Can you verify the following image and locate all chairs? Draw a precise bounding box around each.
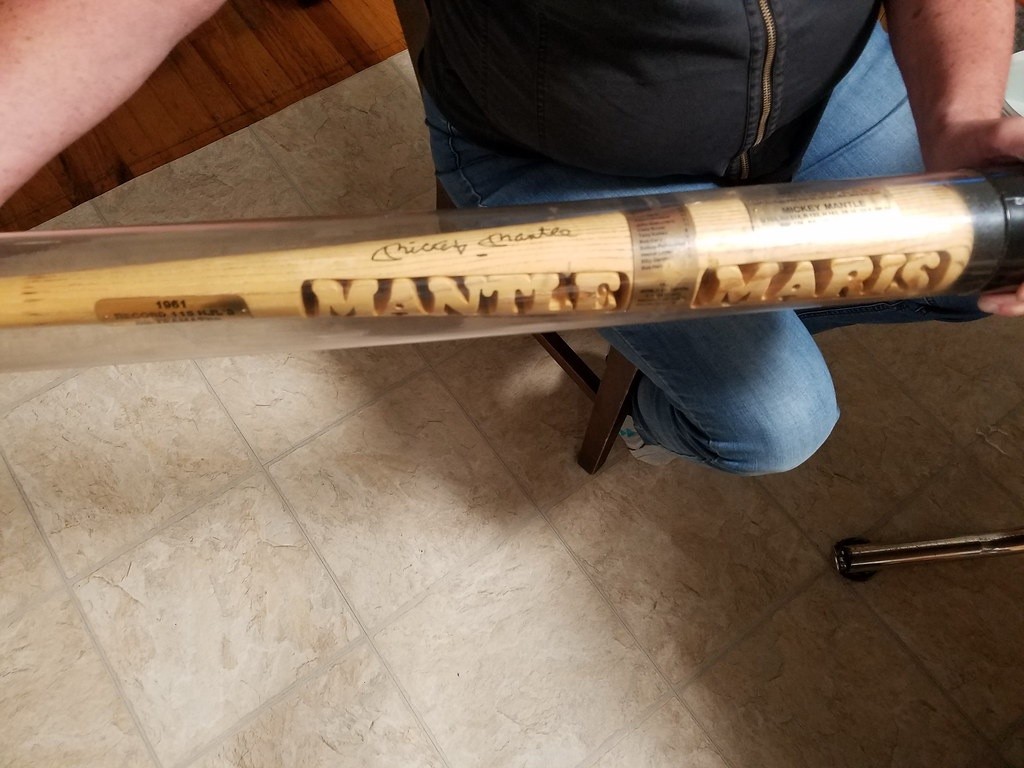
[393,0,646,475]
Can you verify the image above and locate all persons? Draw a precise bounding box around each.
[0,0,1024,476]
